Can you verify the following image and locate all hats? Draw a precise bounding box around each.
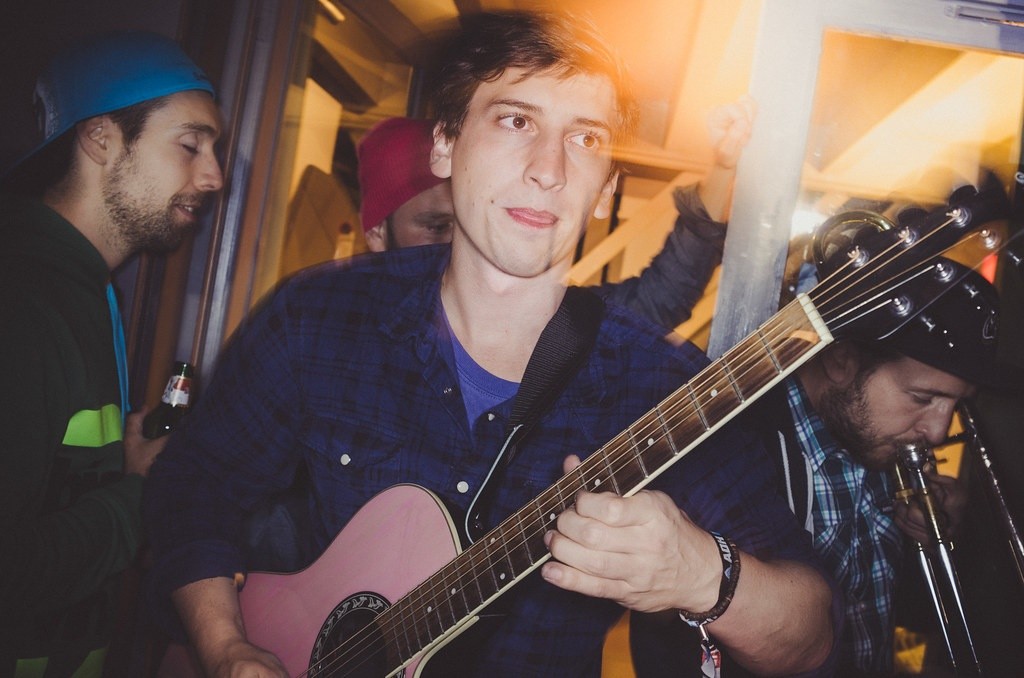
[2,28,218,178]
[850,254,1017,397]
[358,115,452,234]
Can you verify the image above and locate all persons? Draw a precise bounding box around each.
[356,100,754,333]
[761,248,1000,677]
[0,32,225,678]
[140,8,861,678]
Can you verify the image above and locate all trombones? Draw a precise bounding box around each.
[812,210,1023,678]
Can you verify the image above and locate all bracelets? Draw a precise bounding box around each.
[677,531,741,620]
[679,529,735,678]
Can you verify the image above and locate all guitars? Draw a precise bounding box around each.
[153,186,1022,678]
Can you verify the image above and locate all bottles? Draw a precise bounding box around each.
[143,361,198,444]
